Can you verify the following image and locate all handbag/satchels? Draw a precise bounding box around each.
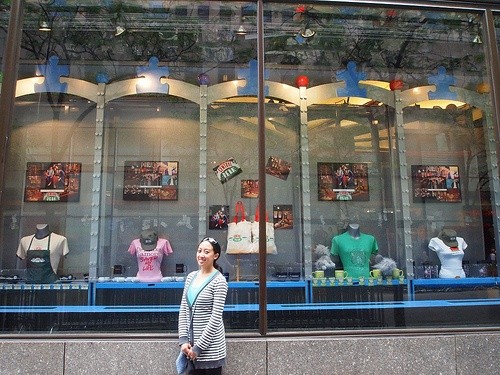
[252,204,277,255]
[226,201,252,254]
[176,351,192,375]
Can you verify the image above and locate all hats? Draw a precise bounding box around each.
[438,229,458,247]
[140,230,158,251]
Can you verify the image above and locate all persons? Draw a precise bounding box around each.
[126,231,174,283]
[279,209,288,222]
[14,224,70,283]
[178,238,228,375]
[243,181,257,193]
[210,210,228,229]
[428,229,469,279]
[136,163,177,186]
[335,165,355,189]
[269,157,286,179]
[328,224,380,278]
[44,165,66,190]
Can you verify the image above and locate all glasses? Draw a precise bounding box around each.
[203,237,219,244]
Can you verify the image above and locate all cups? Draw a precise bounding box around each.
[312,271,324,279]
[392,269,403,279]
[335,271,348,280]
[369,269,381,278]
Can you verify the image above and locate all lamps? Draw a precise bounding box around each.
[38,22,51,31]
[472,35,483,44]
[114,26,126,37]
[301,28,316,38]
[236,25,247,35]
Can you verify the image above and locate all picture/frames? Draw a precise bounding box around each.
[123,161,180,200]
[411,164,463,203]
[24,162,82,203]
[316,161,370,201]
